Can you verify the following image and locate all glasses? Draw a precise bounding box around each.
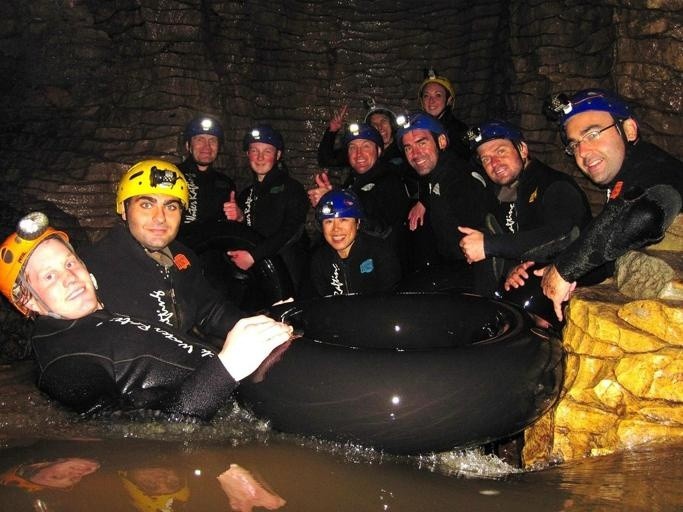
[564,117,624,157]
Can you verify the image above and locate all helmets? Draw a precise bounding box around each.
[181,115,224,145]
[556,88,640,152]
[313,188,364,222]
[0,224,72,322]
[243,125,281,151]
[362,105,398,123]
[343,123,384,153]
[397,113,446,152]
[418,75,457,107]
[461,122,522,160]
[115,159,189,220]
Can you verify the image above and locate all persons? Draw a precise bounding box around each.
[307,77,535,291]
[176,115,310,270]
[504,88,682,331]
[83,161,207,347]
[457,122,595,313]
[1,440,108,497]
[0,210,293,427]
[292,187,412,352]
[114,438,285,511]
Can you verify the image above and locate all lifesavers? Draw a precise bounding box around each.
[241,290,563,456]
